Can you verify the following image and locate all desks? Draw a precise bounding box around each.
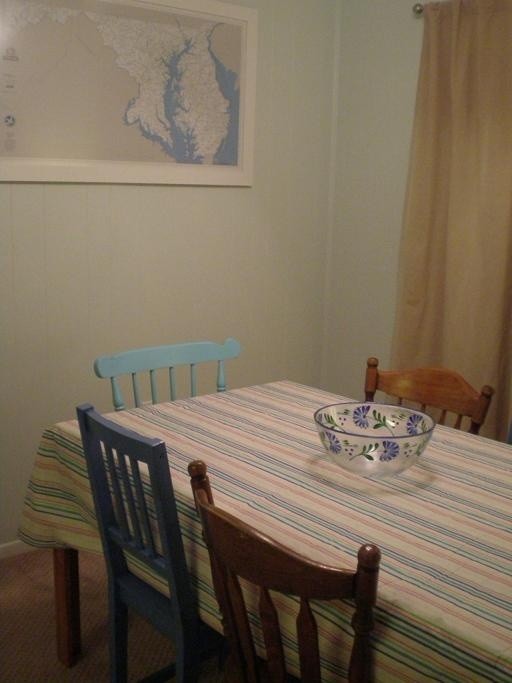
[17,380,512,682]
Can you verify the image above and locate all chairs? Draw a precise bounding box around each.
[365,357,494,434]
[94,337,241,411]
[76,401,230,683]
[188,459,381,683]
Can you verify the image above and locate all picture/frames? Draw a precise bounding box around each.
[1,0,261,187]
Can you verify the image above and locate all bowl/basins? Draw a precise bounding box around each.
[312,398,437,480]
